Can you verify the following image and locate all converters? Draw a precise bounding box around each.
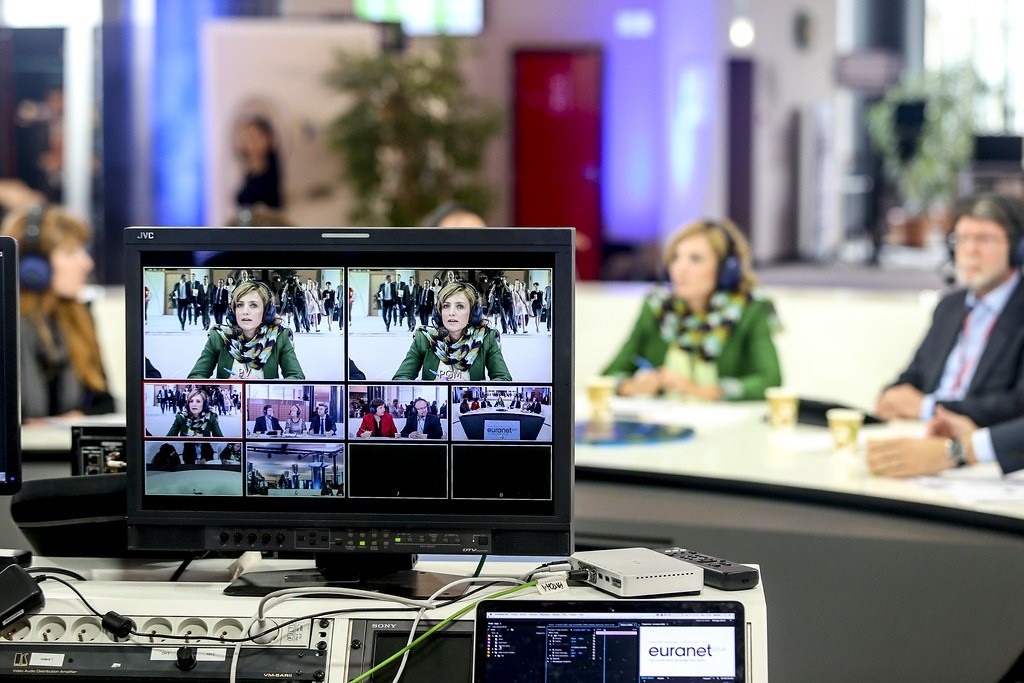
[0,563,45,633]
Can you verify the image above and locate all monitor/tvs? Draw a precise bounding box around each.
[123,225,574,602]
[471,599,747,683]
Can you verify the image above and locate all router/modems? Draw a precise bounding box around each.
[569,546,704,598]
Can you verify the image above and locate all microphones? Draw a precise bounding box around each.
[946,276,955,285]
[438,329,448,337]
[187,412,193,418]
[233,328,242,336]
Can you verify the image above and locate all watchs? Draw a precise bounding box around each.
[943,436,971,469]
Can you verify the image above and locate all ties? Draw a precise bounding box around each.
[321,417,325,435]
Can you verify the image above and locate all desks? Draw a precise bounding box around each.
[10,395,1022,681]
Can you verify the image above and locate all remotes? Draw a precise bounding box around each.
[651,545,759,592]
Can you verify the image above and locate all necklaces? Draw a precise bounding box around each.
[952,302,997,396]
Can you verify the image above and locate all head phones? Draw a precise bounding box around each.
[263,405,274,415]
[225,279,277,328]
[18,201,57,294]
[946,190,1023,267]
[184,389,210,412]
[369,398,385,414]
[432,281,483,328]
[659,218,741,294]
[288,406,300,416]
[412,399,429,415]
[315,404,328,414]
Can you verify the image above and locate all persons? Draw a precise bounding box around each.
[598,214,789,404]
[419,201,491,230]
[862,399,1024,480]
[143,264,553,499]
[0,197,120,423]
[873,190,1024,425]
[226,115,291,228]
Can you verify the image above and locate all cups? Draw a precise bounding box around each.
[395,433,401,439]
[326,432,330,437]
[303,431,307,436]
[825,409,865,448]
[421,434,427,439]
[364,432,371,438]
[587,410,614,439]
[765,386,799,426]
[586,376,618,409]
[256,431,261,437]
[277,430,281,435]
